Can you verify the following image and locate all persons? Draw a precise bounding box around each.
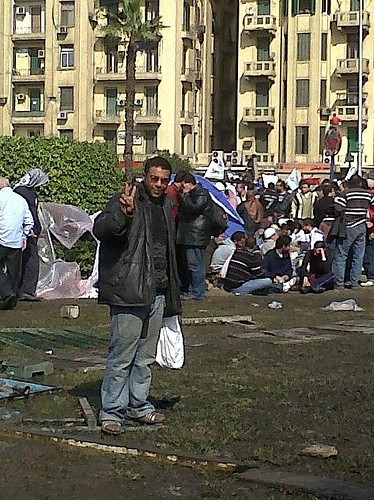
[164,170,374,295]
[92,157,183,434]
[0,176,34,310]
[12,168,48,302]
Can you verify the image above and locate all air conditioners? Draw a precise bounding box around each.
[57,25,67,34]
[117,130,125,145]
[322,149,332,165]
[231,151,242,166]
[17,92,26,100]
[38,49,45,58]
[116,99,126,107]
[16,6,26,16]
[133,130,142,145]
[321,106,334,116]
[57,111,68,120]
[211,150,224,167]
[133,98,143,107]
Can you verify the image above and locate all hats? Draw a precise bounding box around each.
[264,228,275,238]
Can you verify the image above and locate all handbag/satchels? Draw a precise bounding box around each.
[156,315,184,369]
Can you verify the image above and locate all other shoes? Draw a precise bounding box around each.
[360,280,374,286]
[18,291,40,300]
[283,277,296,293]
[179,295,192,300]
[0,294,17,310]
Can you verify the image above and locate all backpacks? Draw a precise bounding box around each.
[192,188,229,237]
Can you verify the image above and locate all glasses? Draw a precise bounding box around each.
[145,172,169,185]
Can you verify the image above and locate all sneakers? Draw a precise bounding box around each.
[101,421,121,434]
[133,412,165,423]
